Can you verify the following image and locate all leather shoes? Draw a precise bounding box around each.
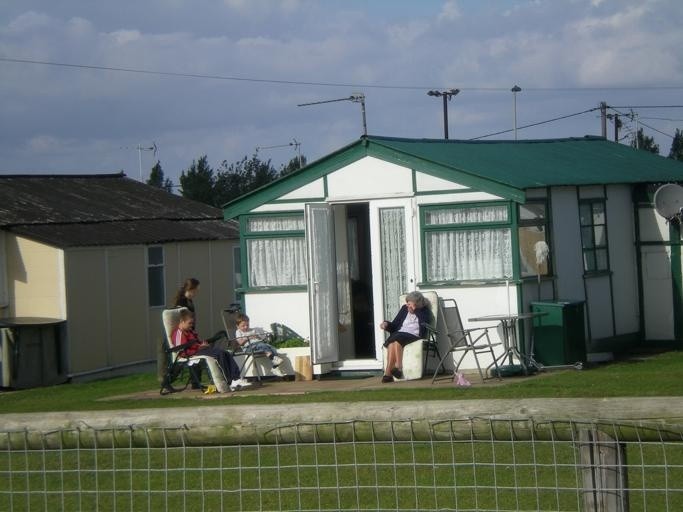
[390,368,401,379]
[381,375,393,384]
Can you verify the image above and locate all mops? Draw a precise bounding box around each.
[532,239,549,302]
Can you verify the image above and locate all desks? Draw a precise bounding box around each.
[468,311,549,378]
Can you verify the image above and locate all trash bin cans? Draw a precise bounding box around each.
[530,298,588,365]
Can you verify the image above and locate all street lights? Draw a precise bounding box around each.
[614,132,635,146]
[511,85,522,139]
[427,88,461,140]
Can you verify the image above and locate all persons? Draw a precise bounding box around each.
[380,290,434,383]
[236,314,286,378]
[172,309,253,391]
[160,278,205,392]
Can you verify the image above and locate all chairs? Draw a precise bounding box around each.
[218,307,274,386]
[159,306,233,394]
[382,291,445,382]
[430,298,503,383]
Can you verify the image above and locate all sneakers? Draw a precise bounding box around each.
[231,378,252,387]
[272,356,284,368]
[230,379,247,392]
